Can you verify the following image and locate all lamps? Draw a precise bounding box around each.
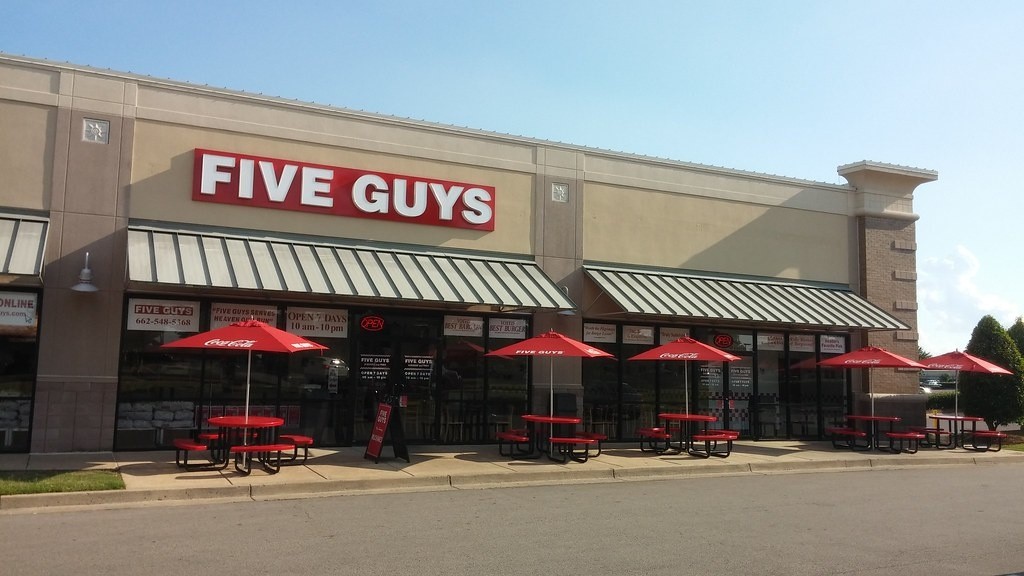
[557,285,576,318]
[69,252,100,292]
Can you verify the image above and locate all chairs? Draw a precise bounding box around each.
[352,395,688,440]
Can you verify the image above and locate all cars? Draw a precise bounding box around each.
[920,387,932,393]
[584,378,643,418]
[303,357,349,384]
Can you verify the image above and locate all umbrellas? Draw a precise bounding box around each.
[419,338,515,363]
[918,348,1014,446]
[817,344,929,450]
[789,357,831,371]
[628,334,742,451]
[485,328,614,459]
[160,314,330,466]
[582,357,620,367]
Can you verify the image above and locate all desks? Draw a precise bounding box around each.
[207,416,285,461]
[846,416,902,446]
[930,415,984,447]
[521,415,581,451]
[659,412,717,450]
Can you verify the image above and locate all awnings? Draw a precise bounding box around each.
[583,266,913,332]
[0,213,51,285]
[127,224,578,313]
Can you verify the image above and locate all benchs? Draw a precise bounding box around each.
[638,426,740,458]
[826,426,1008,455]
[497,429,609,464]
[174,433,313,478]
